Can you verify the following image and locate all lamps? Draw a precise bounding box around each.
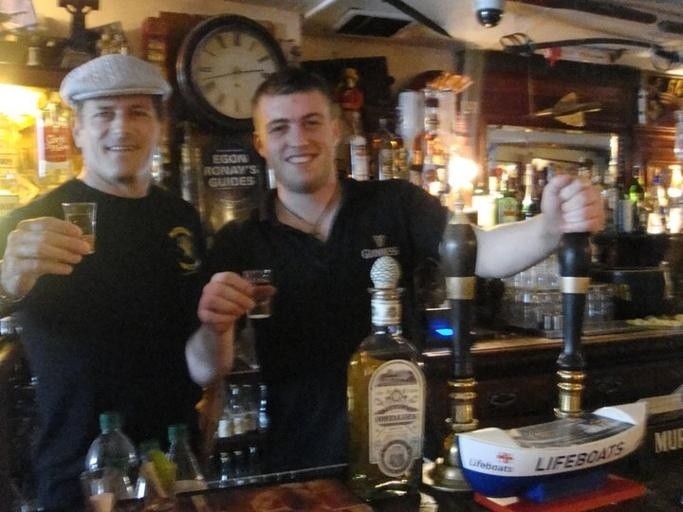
[502,38,682,74]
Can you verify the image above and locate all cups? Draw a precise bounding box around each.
[499,253,614,331]
[58,200,99,256]
[240,267,274,320]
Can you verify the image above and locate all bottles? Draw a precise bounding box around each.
[211,382,270,491]
[164,423,209,496]
[407,150,424,187]
[613,163,683,235]
[79,410,145,503]
[344,254,430,505]
[490,163,543,226]
[342,110,372,183]
[369,117,410,184]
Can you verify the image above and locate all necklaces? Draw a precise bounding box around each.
[275,182,338,239]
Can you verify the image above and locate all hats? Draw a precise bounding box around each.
[59,54,174,109]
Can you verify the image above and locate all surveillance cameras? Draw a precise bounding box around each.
[474,0,505,28]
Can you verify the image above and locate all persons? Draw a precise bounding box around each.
[0,54,228,512]
[169,67,606,470]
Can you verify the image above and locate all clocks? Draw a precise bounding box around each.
[175,14,282,130]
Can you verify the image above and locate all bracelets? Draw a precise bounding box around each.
[0,261,27,307]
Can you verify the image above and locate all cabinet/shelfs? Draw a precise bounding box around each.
[421,318,683,460]
